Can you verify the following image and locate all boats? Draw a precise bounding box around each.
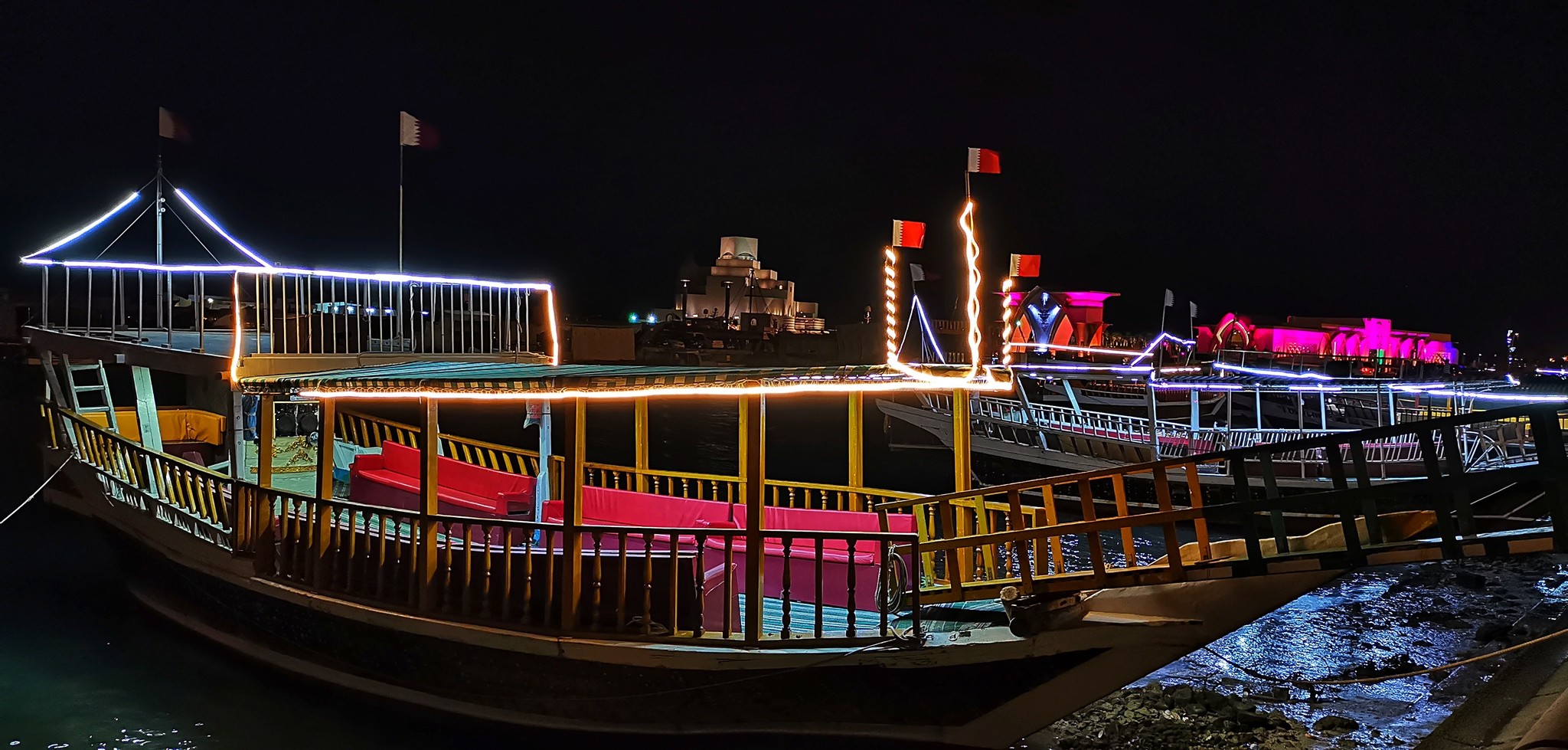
[0,135,1568,750]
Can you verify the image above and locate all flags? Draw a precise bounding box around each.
[1009,254,1041,277]
[968,148,1001,174]
[892,220,926,249]
[401,110,440,149]
[1190,301,1208,319]
[157,107,191,142]
[1165,289,1189,312]
[909,262,940,282]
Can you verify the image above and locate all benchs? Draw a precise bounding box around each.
[82,409,226,446]
[349,440,537,545]
[541,486,916,613]
